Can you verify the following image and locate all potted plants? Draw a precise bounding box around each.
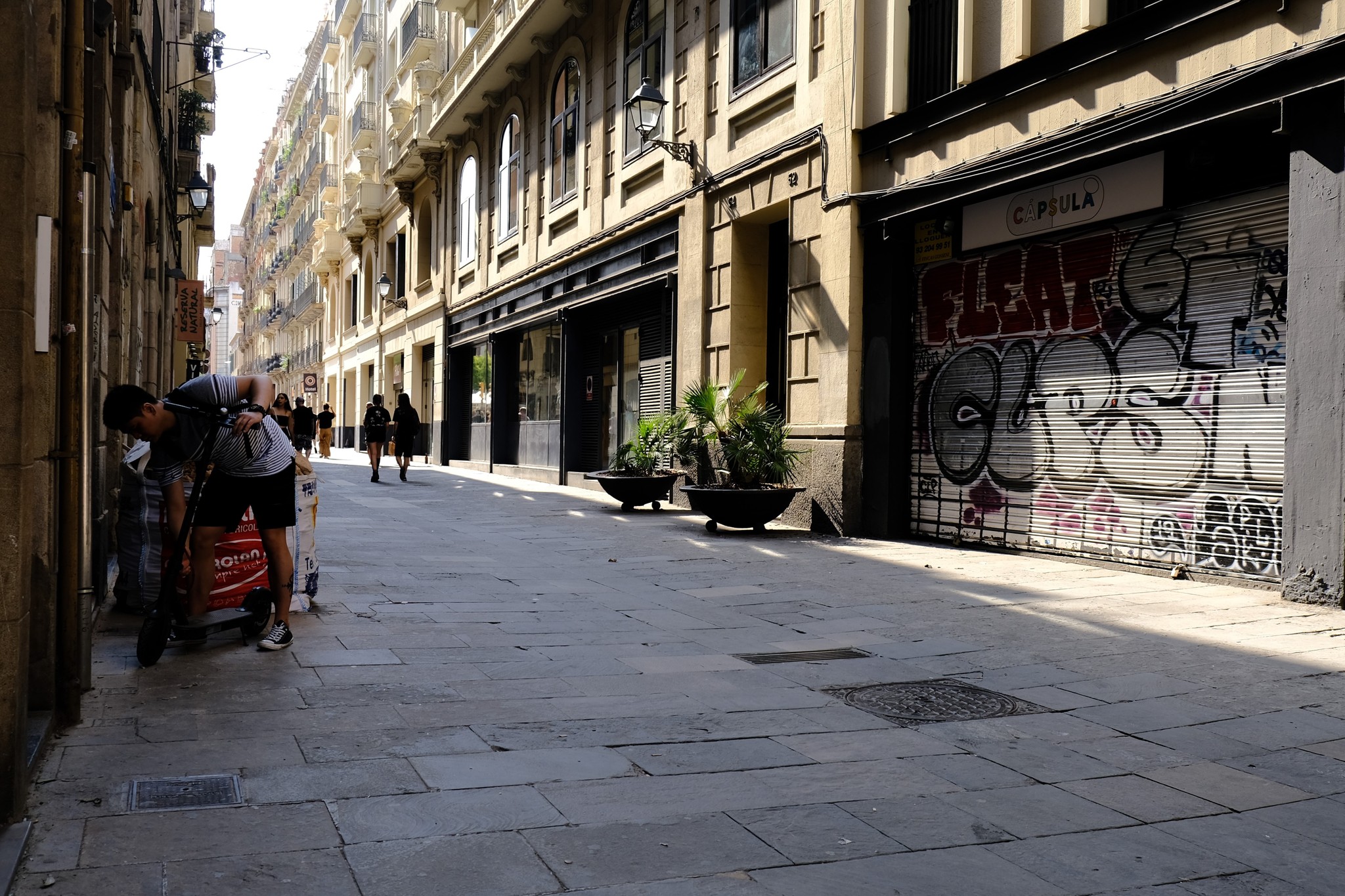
[284,240,302,267]
[173,86,209,159]
[250,268,273,327]
[585,414,680,522]
[195,23,224,79]
[676,374,809,542]
[244,95,306,235]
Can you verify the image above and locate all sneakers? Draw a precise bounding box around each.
[257,619,294,650]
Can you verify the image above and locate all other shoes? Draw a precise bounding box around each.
[324,457,329,459]
[320,455,324,458]
[400,466,407,481]
[371,470,379,482]
[314,446,317,453]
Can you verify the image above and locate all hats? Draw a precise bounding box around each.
[365,401,373,406]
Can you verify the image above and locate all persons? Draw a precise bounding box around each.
[518,406,531,423]
[391,392,420,481]
[290,396,314,462]
[362,401,380,464]
[471,406,492,424]
[101,371,300,650]
[305,405,318,454]
[315,404,336,458]
[267,393,295,449]
[364,393,392,482]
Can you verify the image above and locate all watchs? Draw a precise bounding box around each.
[247,403,267,419]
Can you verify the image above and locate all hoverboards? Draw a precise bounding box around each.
[135,400,274,669]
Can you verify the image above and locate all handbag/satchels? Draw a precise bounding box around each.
[388,440,395,456]
[315,434,319,442]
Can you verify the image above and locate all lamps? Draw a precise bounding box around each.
[188,341,199,358]
[204,306,224,331]
[172,169,213,230]
[373,268,412,312]
[621,72,696,179]
